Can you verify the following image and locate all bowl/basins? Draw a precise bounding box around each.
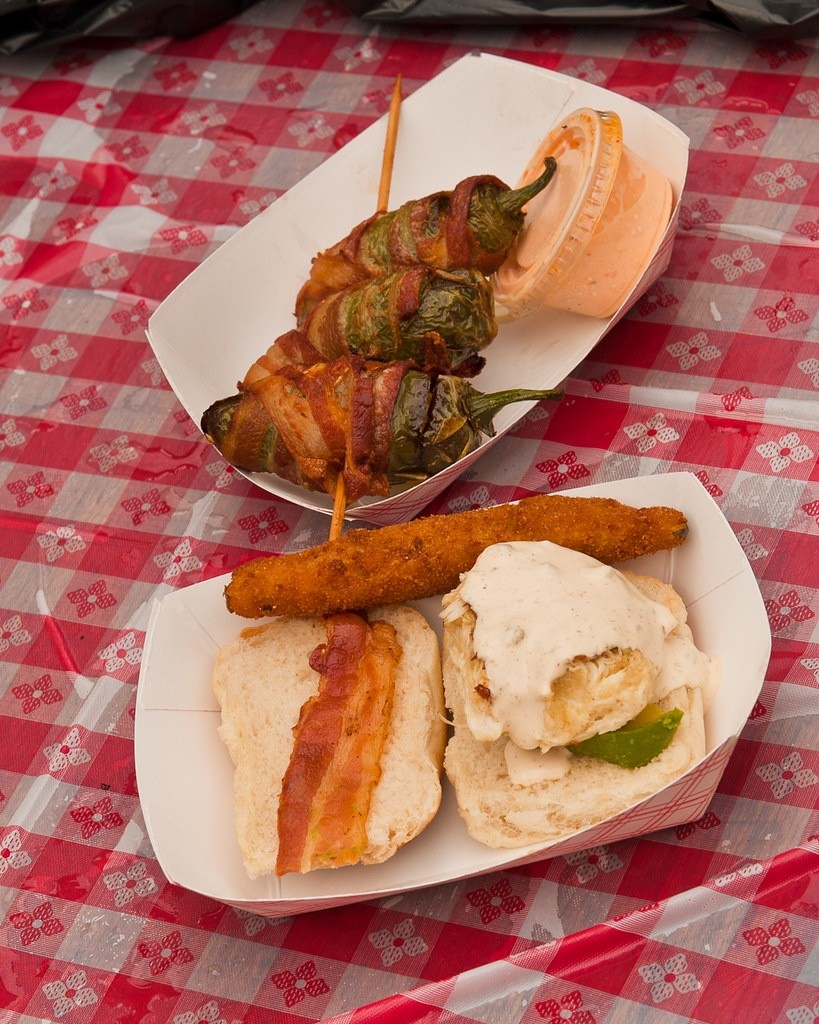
[489,108,672,324]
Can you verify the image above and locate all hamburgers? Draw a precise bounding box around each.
[213,540,705,880]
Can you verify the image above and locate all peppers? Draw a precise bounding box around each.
[197,154,567,488]
